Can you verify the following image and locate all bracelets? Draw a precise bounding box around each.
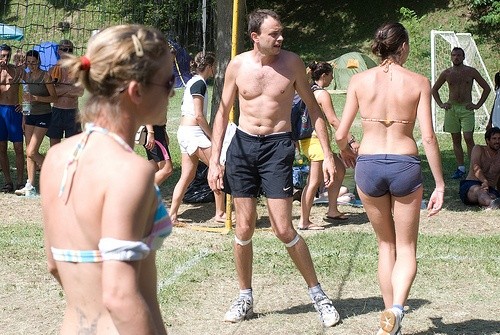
[434,188,444,192]
[148,131,154,134]
[348,135,356,144]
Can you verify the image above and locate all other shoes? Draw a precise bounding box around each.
[15,179,36,196]
[451,169,466,180]
[375,306,404,334]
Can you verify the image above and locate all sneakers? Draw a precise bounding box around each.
[224,296,254,323]
[312,294,340,327]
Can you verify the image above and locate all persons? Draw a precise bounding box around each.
[39,25,174,335]
[486,71,500,131]
[141,122,173,187]
[335,21,445,335]
[298,61,350,230]
[432,47,491,179]
[167,50,236,226]
[207,9,341,329]
[0,40,85,196]
[460,127,500,210]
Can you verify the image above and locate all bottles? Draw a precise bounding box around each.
[292,148,310,166]
[301,114,308,126]
[22,96,31,116]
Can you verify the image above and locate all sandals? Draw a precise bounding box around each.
[17,183,25,190]
[1,183,14,192]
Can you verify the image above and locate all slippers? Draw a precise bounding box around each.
[323,213,350,221]
[297,221,325,230]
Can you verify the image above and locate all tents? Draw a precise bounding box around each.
[324,52,377,95]
[24,42,61,73]
[168,39,192,88]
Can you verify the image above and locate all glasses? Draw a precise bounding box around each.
[1,54,11,58]
[145,74,176,97]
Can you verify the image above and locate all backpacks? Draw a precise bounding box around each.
[291,84,318,141]
